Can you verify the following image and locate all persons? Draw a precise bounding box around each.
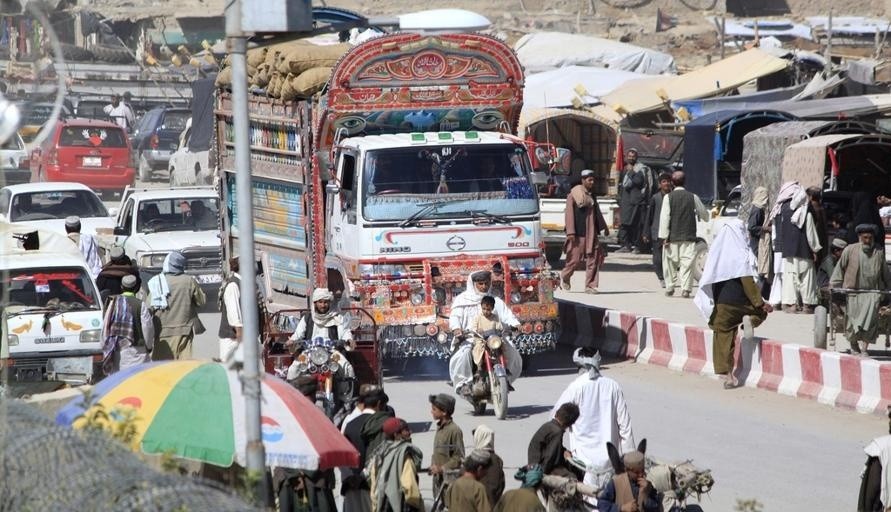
[748,181,891,359]
[95,247,142,308]
[283,288,359,424]
[139,252,205,360]
[693,217,773,388]
[218,257,242,361]
[122,90,136,134]
[66,216,102,284]
[102,92,135,138]
[137,204,164,233]
[195,385,663,511]
[469,156,507,194]
[372,153,401,193]
[187,200,216,225]
[615,148,710,298]
[449,270,523,400]
[559,170,609,294]
[551,345,635,510]
[101,275,155,377]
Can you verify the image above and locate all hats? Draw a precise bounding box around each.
[360,384,407,436]
[430,394,454,413]
[65,216,81,229]
[624,452,644,469]
[120,275,137,289]
[229,256,239,271]
[629,148,637,152]
[471,449,490,462]
[659,171,685,185]
[832,224,878,248]
[581,169,594,177]
[110,247,124,260]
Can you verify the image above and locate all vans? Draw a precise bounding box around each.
[1,232,118,390]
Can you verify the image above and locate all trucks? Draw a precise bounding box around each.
[517,104,624,271]
[212,74,563,374]
[614,98,891,313]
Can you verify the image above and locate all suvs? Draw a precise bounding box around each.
[129,103,193,183]
[166,115,213,185]
[96,184,221,306]
[73,93,133,132]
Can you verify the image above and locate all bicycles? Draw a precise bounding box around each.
[417,467,462,512]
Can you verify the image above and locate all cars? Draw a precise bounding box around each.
[32,119,137,199]
[0,182,119,248]
[0,126,31,184]
[14,101,77,151]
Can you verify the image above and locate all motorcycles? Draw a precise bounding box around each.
[451,324,522,420]
[261,307,387,422]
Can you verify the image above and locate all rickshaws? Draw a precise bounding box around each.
[812,286,887,358]
